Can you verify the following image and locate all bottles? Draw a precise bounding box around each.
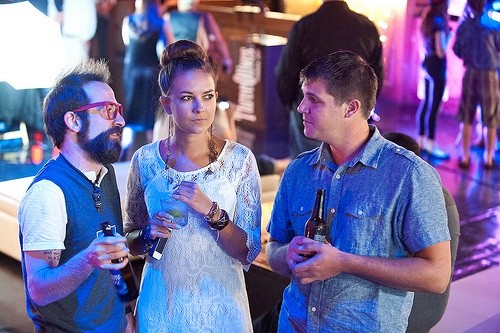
[101,221,139,302]
[303,188,327,257]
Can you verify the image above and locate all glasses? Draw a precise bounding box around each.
[73,102,124,119]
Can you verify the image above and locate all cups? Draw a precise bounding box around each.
[160,197,189,228]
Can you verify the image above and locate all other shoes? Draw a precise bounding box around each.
[419,148,449,165]
[457,150,470,168]
[482,156,494,169]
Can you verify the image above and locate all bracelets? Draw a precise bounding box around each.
[137,225,154,255]
[204,201,230,242]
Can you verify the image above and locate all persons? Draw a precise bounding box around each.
[154,0,233,143]
[382,129,460,333]
[122,0,166,165]
[275,0,384,162]
[455,1,499,148]
[17,58,133,333]
[415,0,449,161]
[120,39,262,332]
[453,0,499,171]
[265,49,453,333]
